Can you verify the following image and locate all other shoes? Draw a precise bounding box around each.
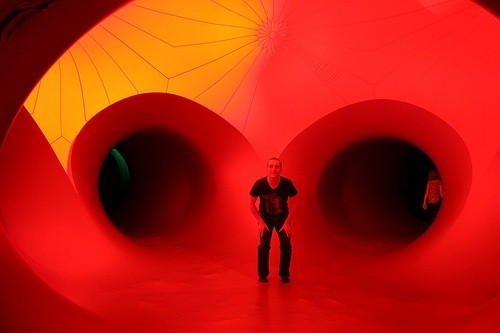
[259,275,269,282]
[280,276,289,283]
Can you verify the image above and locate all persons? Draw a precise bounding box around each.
[423,168,444,219]
[248,158,298,283]
